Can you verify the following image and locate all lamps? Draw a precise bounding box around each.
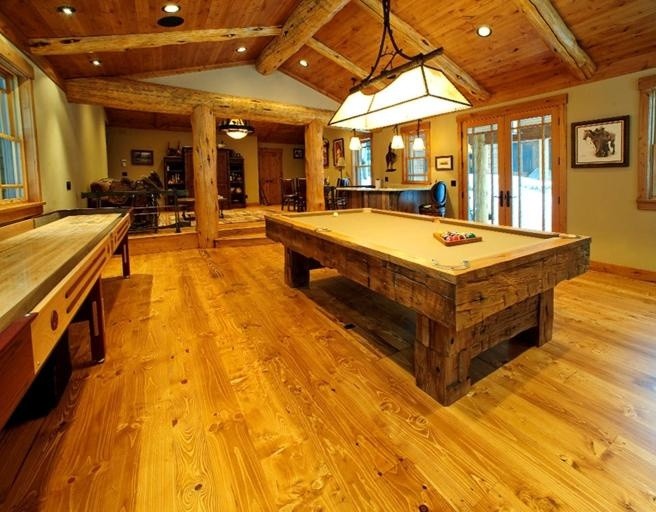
[219,119,255,139]
[328,0,473,150]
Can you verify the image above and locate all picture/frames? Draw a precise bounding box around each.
[435,155,453,171]
[323,143,329,166]
[293,148,303,159]
[333,138,344,166]
[571,114,631,168]
[131,149,153,166]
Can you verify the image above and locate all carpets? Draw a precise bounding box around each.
[152,211,265,226]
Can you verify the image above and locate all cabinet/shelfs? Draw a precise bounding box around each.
[165,146,247,207]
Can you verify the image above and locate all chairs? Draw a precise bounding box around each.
[418,182,447,217]
[280,178,349,212]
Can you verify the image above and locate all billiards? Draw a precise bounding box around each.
[334,211,339,217]
[440,231,476,241]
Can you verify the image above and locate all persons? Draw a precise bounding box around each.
[336,142,342,162]
[602,132,612,158]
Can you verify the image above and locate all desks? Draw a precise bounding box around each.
[264,207,590,407]
[0,205,133,432]
[178,196,225,218]
[337,187,431,213]
